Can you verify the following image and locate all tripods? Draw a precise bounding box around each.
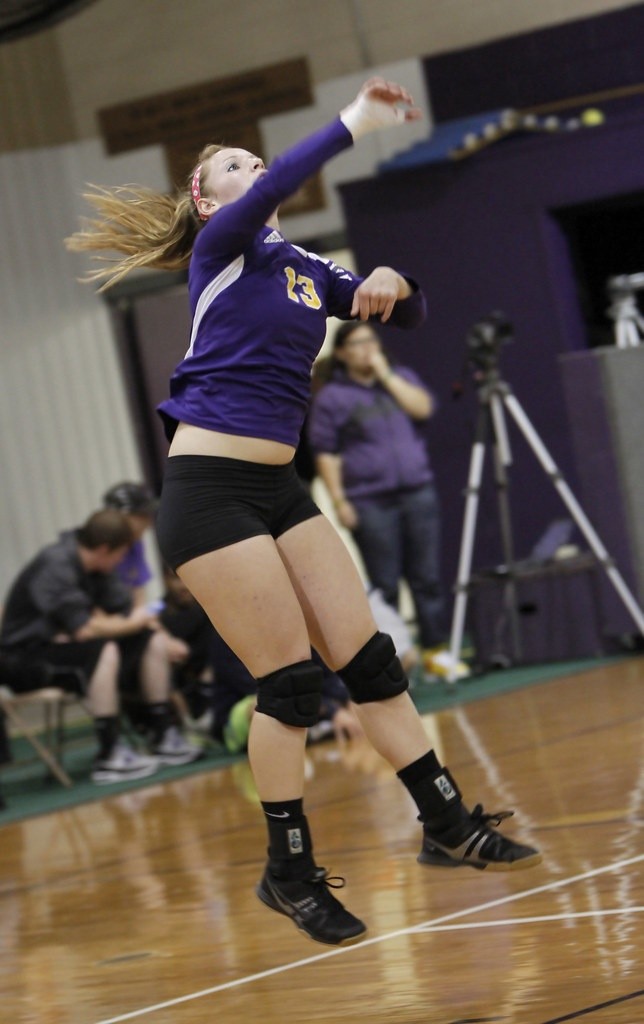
[442,363,644,694]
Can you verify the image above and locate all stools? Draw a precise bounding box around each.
[0,686,140,792]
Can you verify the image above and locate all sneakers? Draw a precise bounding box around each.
[421,651,471,685]
[255,846,367,947]
[91,744,159,786]
[147,743,206,767]
[416,803,543,871]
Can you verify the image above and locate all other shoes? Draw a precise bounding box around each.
[223,694,258,752]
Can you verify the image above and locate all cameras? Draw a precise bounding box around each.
[457,311,516,363]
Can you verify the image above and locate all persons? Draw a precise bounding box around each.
[309,319,472,683]
[65,78,543,947]
[0,480,363,786]
